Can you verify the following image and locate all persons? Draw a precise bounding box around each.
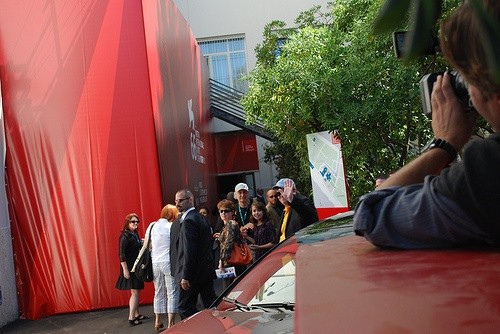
[352,0,500,252]
[116,178,317,330]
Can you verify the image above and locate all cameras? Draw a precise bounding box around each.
[419,71,474,116]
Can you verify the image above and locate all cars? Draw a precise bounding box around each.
[163,208,500,334]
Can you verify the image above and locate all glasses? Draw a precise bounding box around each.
[177,197,188,203]
[131,220,139,224]
[270,195,277,198]
[276,191,282,196]
[219,209,232,213]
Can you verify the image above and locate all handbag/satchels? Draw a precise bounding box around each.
[134,248,154,282]
[226,239,252,266]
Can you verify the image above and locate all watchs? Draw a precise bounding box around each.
[421,137,458,164]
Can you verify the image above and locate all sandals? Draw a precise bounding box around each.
[128,319,143,326]
[134,314,151,320]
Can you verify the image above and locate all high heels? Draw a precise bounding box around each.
[155,322,164,331]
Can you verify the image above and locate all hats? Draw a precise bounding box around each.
[272,178,296,190]
[234,183,250,192]
